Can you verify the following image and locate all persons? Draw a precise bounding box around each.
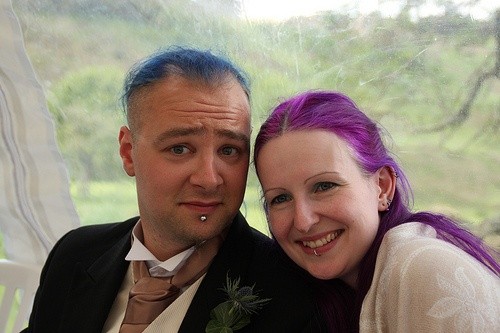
[253,86,500,333]
[19,47,351,333]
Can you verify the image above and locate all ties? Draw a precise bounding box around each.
[119,235,221,333]
